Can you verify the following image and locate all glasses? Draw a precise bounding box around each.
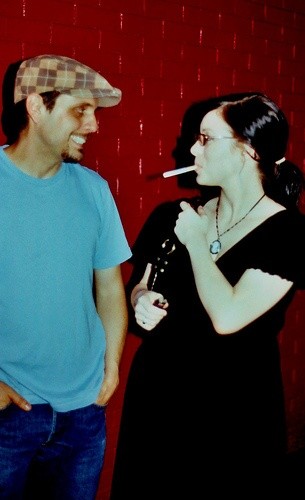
[194,134,241,146]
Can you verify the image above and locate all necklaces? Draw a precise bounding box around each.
[208,191,266,255]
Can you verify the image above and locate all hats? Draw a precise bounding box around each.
[14,55,122,107]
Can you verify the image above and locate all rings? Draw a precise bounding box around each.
[141,317,147,325]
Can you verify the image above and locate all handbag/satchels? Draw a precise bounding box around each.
[124,238,175,340]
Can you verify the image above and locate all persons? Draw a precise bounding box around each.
[109,92,305,500]
[0,54,134,500]
